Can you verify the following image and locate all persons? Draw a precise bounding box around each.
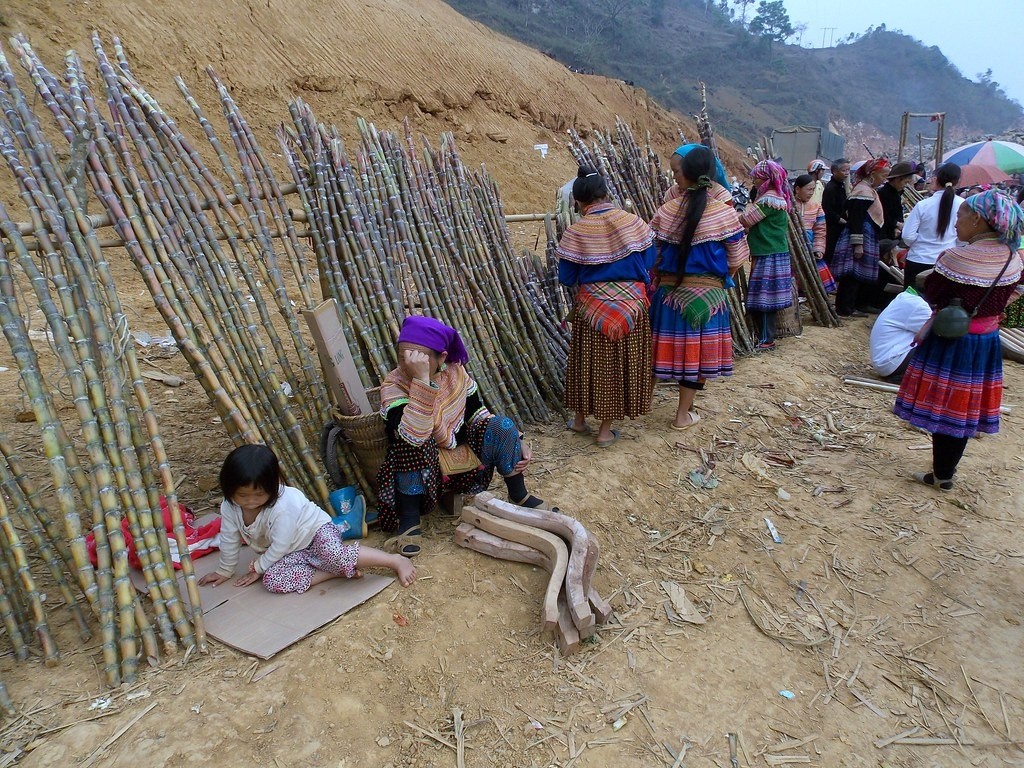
[542,51,557,60]
[901,190,1024,491]
[739,160,793,349]
[621,79,635,86]
[567,62,595,75]
[556,166,658,447]
[796,156,1024,382]
[197,444,416,594]
[380,316,561,556]
[647,144,750,429]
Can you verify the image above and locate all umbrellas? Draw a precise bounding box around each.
[925,137,1024,174]
[923,158,1013,189]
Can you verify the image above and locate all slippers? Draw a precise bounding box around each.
[597,429,620,448]
[567,419,590,435]
[670,411,700,431]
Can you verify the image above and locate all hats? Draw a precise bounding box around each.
[886,161,918,179]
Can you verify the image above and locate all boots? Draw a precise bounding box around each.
[328,485,379,525]
[329,495,368,540]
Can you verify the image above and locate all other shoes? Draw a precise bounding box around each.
[849,310,870,317]
[759,341,775,351]
[857,305,881,315]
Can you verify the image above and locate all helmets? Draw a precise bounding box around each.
[807,159,831,174]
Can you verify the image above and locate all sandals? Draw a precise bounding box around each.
[383,518,423,556]
[913,471,953,492]
[509,492,564,517]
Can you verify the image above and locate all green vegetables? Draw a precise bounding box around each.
[1004,294,1024,329]
